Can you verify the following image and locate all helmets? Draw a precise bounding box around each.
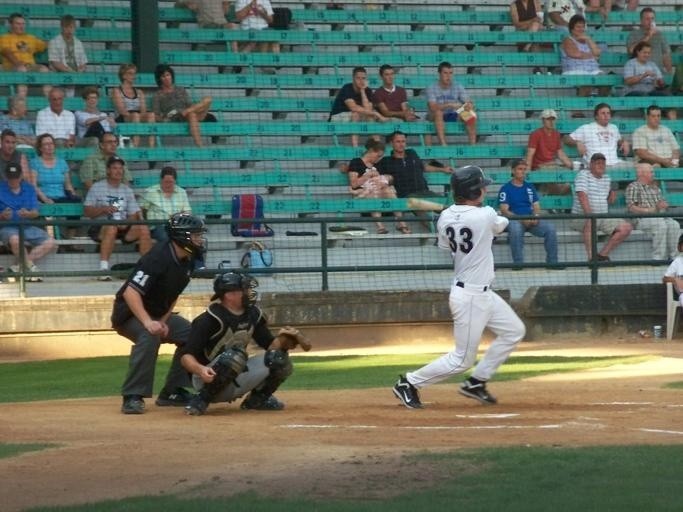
[451,165,493,200]
[210,273,259,311]
[164,213,209,257]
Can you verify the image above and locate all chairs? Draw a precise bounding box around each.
[664,277,682,340]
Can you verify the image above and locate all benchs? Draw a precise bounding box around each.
[0,0,682,246]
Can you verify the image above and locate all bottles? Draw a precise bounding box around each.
[113,201,120,220]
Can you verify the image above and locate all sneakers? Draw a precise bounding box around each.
[185,392,208,416]
[156,388,193,406]
[510,255,614,271]
[8,261,44,282]
[392,374,423,408]
[240,390,284,409]
[96,265,113,281]
[121,394,145,414]
[458,376,497,405]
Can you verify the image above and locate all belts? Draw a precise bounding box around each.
[455,282,487,290]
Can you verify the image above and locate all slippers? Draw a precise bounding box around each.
[377,228,389,240]
[397,225,411,234]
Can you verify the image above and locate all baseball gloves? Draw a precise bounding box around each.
[275,325,311,351]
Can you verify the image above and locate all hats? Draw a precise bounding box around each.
[511,159,528,177]
[108,156,125,165]
[5,162,22,179]
[540,109,558,121]
[592,152,605,161]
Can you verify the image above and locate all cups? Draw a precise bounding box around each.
[652,326,662,336]
[571,161,581,171]
[536,12,543,23]
[670,159,679,167]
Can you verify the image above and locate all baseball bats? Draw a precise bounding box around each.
[408,197,450,212]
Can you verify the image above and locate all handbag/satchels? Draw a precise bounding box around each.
[248,250,273,268]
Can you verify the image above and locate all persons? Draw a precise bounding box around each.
[511,0,637,96]
[177,270,313,416]
[624,7,682,119]
[1,13,212,284]
[389,164,527,409]
[498,103,682,271]
[329,61,475,145]
[186,1,278,75]
[662,231,683,311]
[337,131,453,234]
[110,210,208,415]
